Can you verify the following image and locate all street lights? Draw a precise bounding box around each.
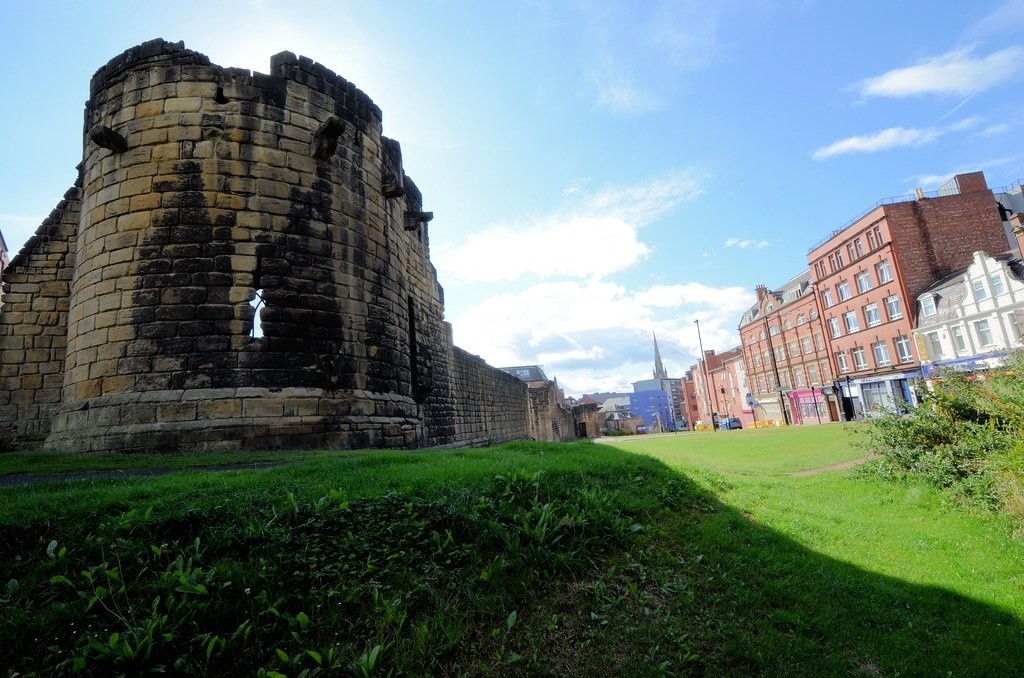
[694,319,717,432]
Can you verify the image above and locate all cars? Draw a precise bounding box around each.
[720,418,743,431]
